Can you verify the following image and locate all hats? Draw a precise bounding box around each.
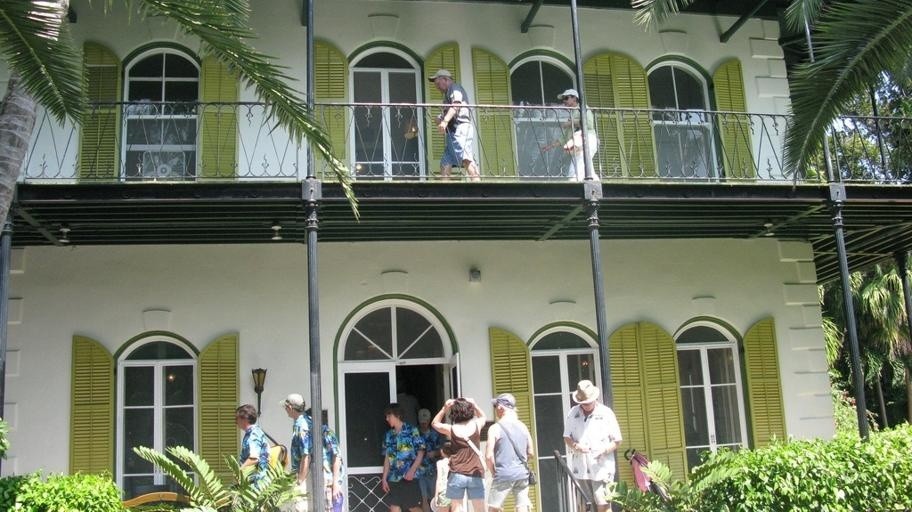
[417,409,432,424]
[557,89,580,100]
[571,379,601,405]
[279,394,307,412]
[428,69,451,81]
[490,392,517,410]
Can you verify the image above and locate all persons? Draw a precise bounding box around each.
[282,393,331,512]
[431,398,488,511]
[382,405,425,511]
[236,405,268,486]
[431,440,468,511]
[485,393,536,511]
[428,69,479,180]
[540,88,600,180]
[416,406,446,511]
[305,409,343,512]
[563,380,622,512]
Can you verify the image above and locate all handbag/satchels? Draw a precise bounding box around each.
[260,428,288,475]
[496,420,537,487]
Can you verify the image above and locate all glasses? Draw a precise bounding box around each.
[563,96,570,101]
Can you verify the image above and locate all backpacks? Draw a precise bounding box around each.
[264,431,289,470]
